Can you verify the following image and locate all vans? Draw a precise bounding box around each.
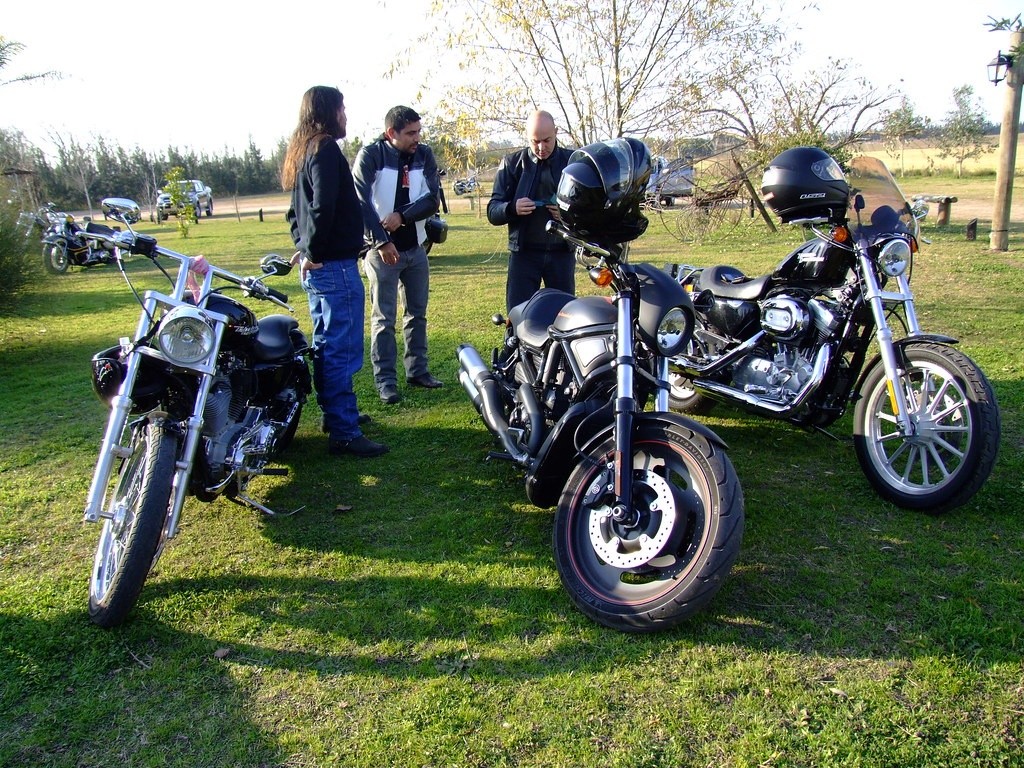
[646,155,694,207]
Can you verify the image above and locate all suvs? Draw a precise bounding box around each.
[156,180,214,220]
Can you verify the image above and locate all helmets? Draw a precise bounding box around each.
[92,340,171,414]
[762,147,849,226]
[555,138,652,243]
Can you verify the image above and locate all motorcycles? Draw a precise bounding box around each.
[455,216,746,634]
[453,176,480,196]
[33,201,121,276]
[668,157,1002,516]
[72,196,317,630]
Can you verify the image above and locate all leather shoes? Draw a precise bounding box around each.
[407,373,444,388]
[321,410,371,433]
[329,435,389,457]
[379,385,399,403]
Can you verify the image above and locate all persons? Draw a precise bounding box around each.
[281,85,389,457]
[350,104,442,403]
[486,111,577,314]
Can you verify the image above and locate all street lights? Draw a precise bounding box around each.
[986,29,1024,251]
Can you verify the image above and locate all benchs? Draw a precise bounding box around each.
[911,193,957,224]
